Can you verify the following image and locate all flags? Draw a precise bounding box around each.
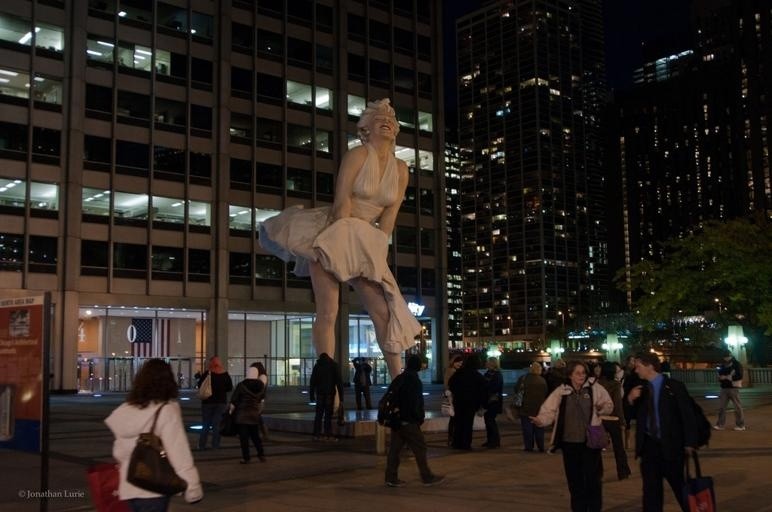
[130,317,171,363]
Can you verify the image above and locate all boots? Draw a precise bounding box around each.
[624,429,631,449]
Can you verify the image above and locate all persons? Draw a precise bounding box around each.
[712,350,749,431]
[193,357,632,479]
[526,360,615,510]
[621,353,713,511]
[375,354,451,488]
[307,351,343,439]
[260,96,426,422]
[106,358,202,512]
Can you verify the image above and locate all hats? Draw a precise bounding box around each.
[407,354,421,371]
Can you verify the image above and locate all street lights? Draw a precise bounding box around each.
[714,297,722,314]
[557,311,565,325]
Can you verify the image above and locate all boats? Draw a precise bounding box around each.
[498,346,551,369]
[561,345,604,359]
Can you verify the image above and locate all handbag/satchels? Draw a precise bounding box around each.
[127,432,187,495]
[196,370,213,400]
[378,392,399,428]
[218,401,237,437]
[514,391,524,407]
[681,477,716,511]
[585,425,610,448]
[441,389,455,417]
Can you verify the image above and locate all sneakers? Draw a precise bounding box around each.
[384,480,407,488]
[713,425,725,430]
[422,475,445,487]
[734,427,745,430]
